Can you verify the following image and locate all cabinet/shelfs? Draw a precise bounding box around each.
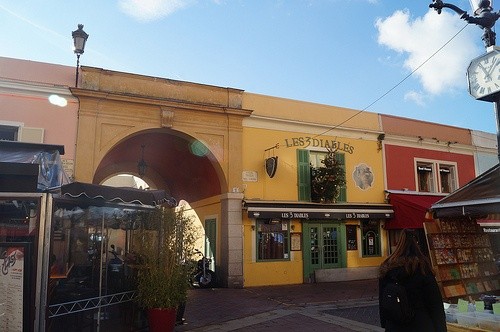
[427,217,500,298]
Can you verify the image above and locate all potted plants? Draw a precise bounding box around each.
[134,203,197,332]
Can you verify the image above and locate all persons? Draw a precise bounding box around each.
[176,300,188,326]
[377,229,447,332]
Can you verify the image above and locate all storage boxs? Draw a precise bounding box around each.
[445,304,500,331]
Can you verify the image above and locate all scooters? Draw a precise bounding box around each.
[176,250,213,293]
[108,242,124,291]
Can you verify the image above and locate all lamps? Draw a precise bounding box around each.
[71,24,89,88]
[138,144,147,179]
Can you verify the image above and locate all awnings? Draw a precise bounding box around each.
[429,163,500,218]
[0,143,65,192]
[59,182,167,208]
[388,192,446,228]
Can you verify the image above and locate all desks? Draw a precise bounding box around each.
[446,322,493,332]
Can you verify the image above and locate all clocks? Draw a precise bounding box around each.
[465,50,500,103]
